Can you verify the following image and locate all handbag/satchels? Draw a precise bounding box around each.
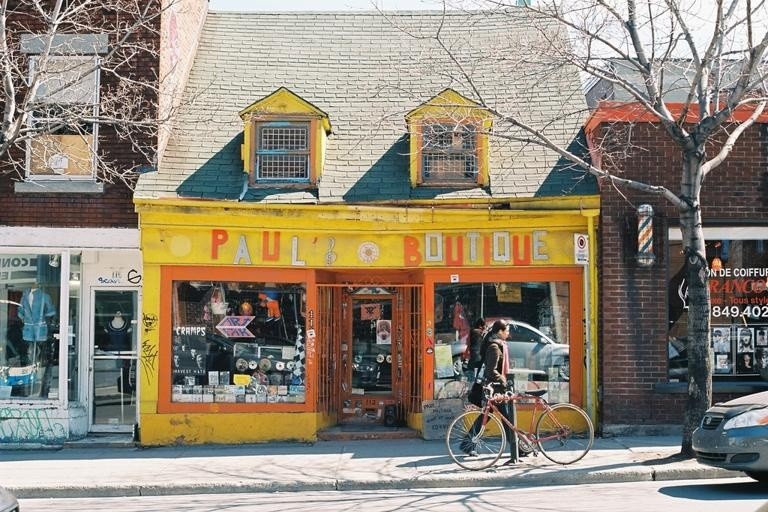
[468,381,486,408]
[462,366,475,377]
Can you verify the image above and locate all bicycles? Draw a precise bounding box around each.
[447,381,593,467]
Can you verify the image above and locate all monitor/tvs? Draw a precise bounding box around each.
[258,347,283,358]
[233,342,259,358]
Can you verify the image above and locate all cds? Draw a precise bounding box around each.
[248,359,296,371]
[354,354,392,363]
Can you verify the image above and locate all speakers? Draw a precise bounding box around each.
[37,254,61,285]
[384,405,404,427]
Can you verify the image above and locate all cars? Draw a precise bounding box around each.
[690,392,768,484]
[170,318,391,393]
[449,320,570,381]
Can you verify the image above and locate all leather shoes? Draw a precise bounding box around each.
[460,445,479,457]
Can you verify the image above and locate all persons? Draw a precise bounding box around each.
[464,317,489,381]
[459,319,533,458]
[713,325,767,374]
[103,310,133,349]
[17,287,57,345]
